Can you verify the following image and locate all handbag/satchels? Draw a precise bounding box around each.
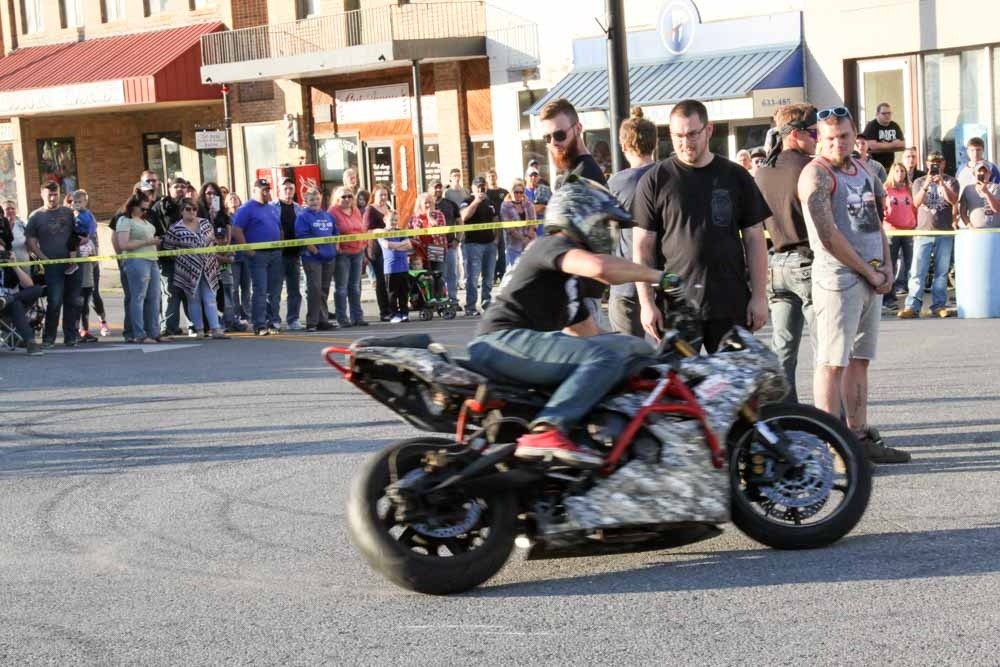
[426,245,445,263]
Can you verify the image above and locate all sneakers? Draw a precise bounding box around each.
[513,428,602,468]
[853,426,910,463]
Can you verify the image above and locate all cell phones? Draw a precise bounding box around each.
[212,197,220,211]
[977,174,984,184]
[481,187,485,192]
[930,164,939,176]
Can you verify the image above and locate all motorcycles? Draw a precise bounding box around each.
[320,277,875,598]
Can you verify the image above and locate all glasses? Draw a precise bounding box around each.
[184,207,198,211]
[543,120,578,144]
[513,190,524,194]
[671,123,708,142]
[138,206,150,212]
[816,107,854,129]
[339,194,352,199]
[798,127,817,134]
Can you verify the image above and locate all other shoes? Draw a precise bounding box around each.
[898,306,920,318]
[124,322,248,344]
[254,320,368,336]
[29,322,112,356]
[933,308,952,318]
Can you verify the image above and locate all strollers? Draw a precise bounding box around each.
[407,240,461,321]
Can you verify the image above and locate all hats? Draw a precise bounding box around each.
[526,167,540,176]
[928,151,946,162]
[254,178,271,189]
[214,227,227,235]
[471,176,489,185]
[975,160,992,172]
[429,180,443,188]
[528,159,540,166]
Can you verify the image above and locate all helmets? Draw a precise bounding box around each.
[544,173,640,255]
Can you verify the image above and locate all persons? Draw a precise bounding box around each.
[885,136,999,319]
[465,174,686,468]
[0,159,552,357]
[536,99,910,464]
